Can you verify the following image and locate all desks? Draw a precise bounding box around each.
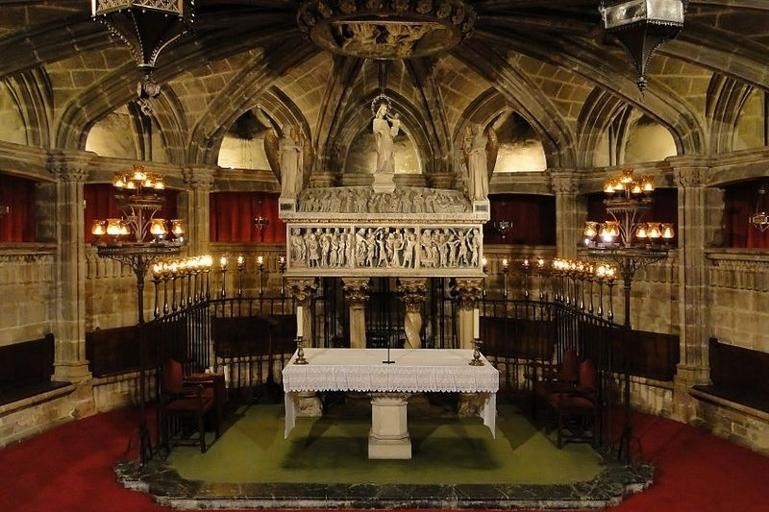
[280,346,505,459]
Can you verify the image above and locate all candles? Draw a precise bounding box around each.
[471,307,482,339]
[295,303,305,339]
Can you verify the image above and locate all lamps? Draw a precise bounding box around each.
[151,253,286,281]
[581,168,672,249]
[476,256,615,280]
[92,168,187,242]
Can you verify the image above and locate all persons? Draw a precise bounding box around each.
[463,123,489,200]
[373,99,397,173]
[386,112,401,138]
[290,189,479,268]
[278,123,303,199]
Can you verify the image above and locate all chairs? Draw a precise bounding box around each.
[154,354,231,458]
[527,343,609,453]
